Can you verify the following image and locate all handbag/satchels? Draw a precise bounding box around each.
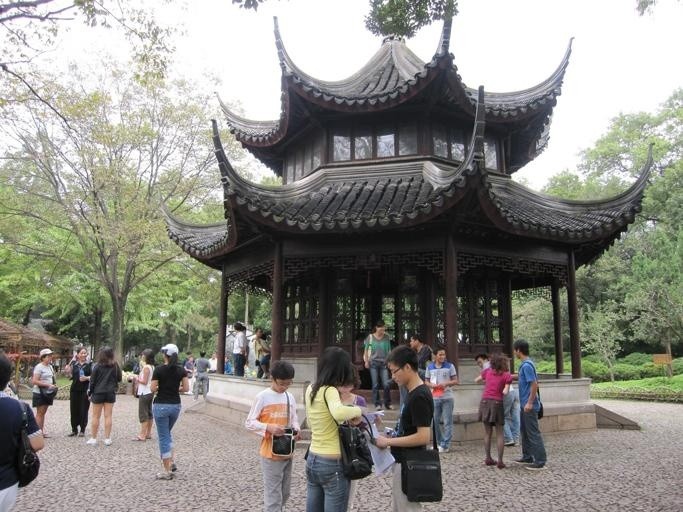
[363,333,373,362]
[38,375,58,401]
[537,401,544,420]
[401,390,443,503]
[323,385,374,482]
[17,400,40,488]
[271,427,296,457]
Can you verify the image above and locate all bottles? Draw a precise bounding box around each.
[79,367,85,377]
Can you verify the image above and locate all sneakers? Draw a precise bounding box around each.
[485,459,506,470]
[525,462,545,470]
[85,437,97,446]
[155,472,173,480]
[375,404,396,411]
[67,431,85,438]
[438,445,450,453]
[131,435,151,442]
[505,437,520,447]
[104,437,113,446]
[171,460,177,472]
[513,457,534,464]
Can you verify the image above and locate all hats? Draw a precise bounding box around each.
[161,343,179,357]
[39,348,55,358]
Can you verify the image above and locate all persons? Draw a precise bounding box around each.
[509,338,547,471]
[148,343,189,481]
[0,350,44,511]
[61,347,93,437]
[131,353,141,398]
[245,360,301,511]
[84,346,121,446]
[181,321,272,402]
[126,348,159,443]
[31,347,57,437]
[473,352,513,469]
[475,351,522,447]
[301,318,460,511]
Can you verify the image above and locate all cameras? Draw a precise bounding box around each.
[384,426,397,439]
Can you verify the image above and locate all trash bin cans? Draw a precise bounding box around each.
[126,377,133,394]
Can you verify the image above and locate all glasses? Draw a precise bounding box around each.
[389,366,402,376]
[274,379,293,387]
